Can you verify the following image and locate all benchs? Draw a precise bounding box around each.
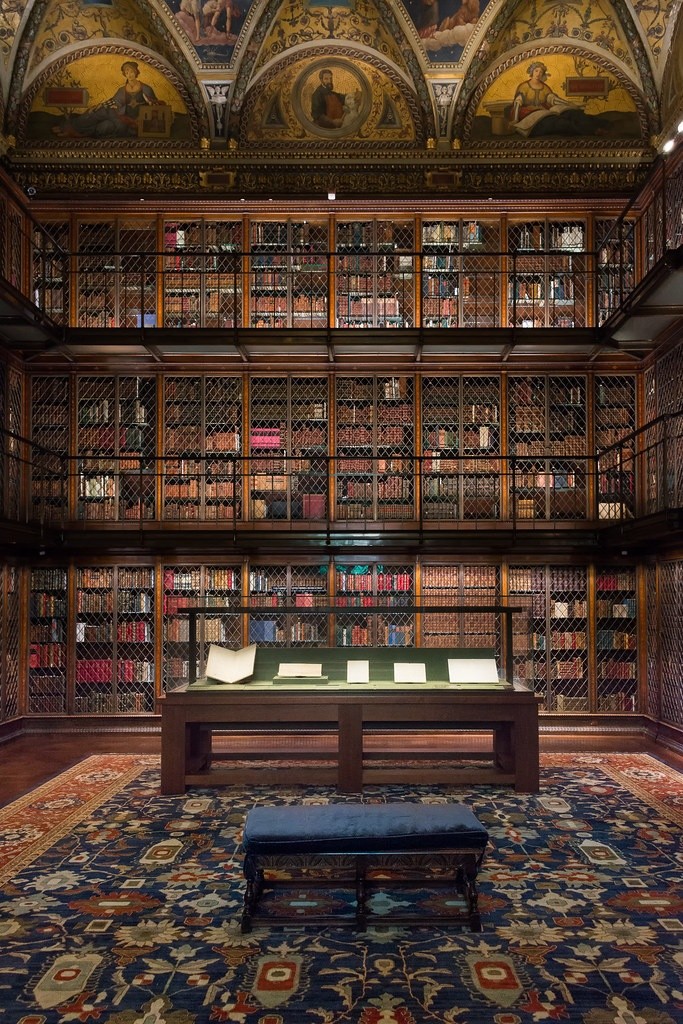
[241,804,489,932]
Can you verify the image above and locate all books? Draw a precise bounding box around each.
[0,568,634,711]
[32,379,635,519]
[45,219,636,326]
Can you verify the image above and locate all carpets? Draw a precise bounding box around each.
[0,753,683,1024]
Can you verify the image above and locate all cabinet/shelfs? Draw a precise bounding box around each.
[0,145,683,756]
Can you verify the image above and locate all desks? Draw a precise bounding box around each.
[156,678,544,794]
